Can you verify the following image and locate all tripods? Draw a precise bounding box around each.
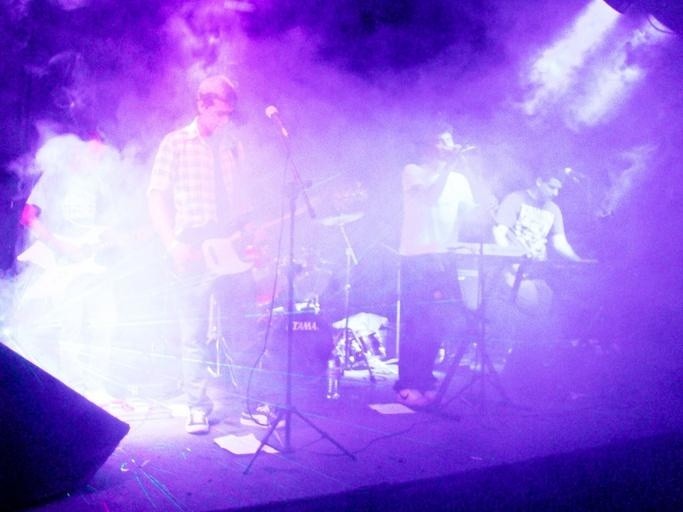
[438,231,512,415]
[242,182,356,476]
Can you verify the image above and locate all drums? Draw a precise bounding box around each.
[264,309,334,379]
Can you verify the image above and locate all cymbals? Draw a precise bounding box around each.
[321,214,363,227]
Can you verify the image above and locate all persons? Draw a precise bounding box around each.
[493,165,582,315]
[147,75,286,433]
[394,121,474,410]
[327,349,344,399]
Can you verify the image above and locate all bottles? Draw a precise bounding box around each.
[326,360,338,398]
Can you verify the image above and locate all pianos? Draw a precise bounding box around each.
[400,252,597,278]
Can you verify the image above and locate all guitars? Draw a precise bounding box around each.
[174,183,367,300]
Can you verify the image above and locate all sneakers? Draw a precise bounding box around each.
[183,405,210,436]
[236,401,286,429]
[423,389,449,404]
[393,388,429,409]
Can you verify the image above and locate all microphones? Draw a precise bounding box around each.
[565,166,591,186]
[266,104,291,152]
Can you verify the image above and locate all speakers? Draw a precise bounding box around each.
[0,343,129,512]
[273,312,340,376]
